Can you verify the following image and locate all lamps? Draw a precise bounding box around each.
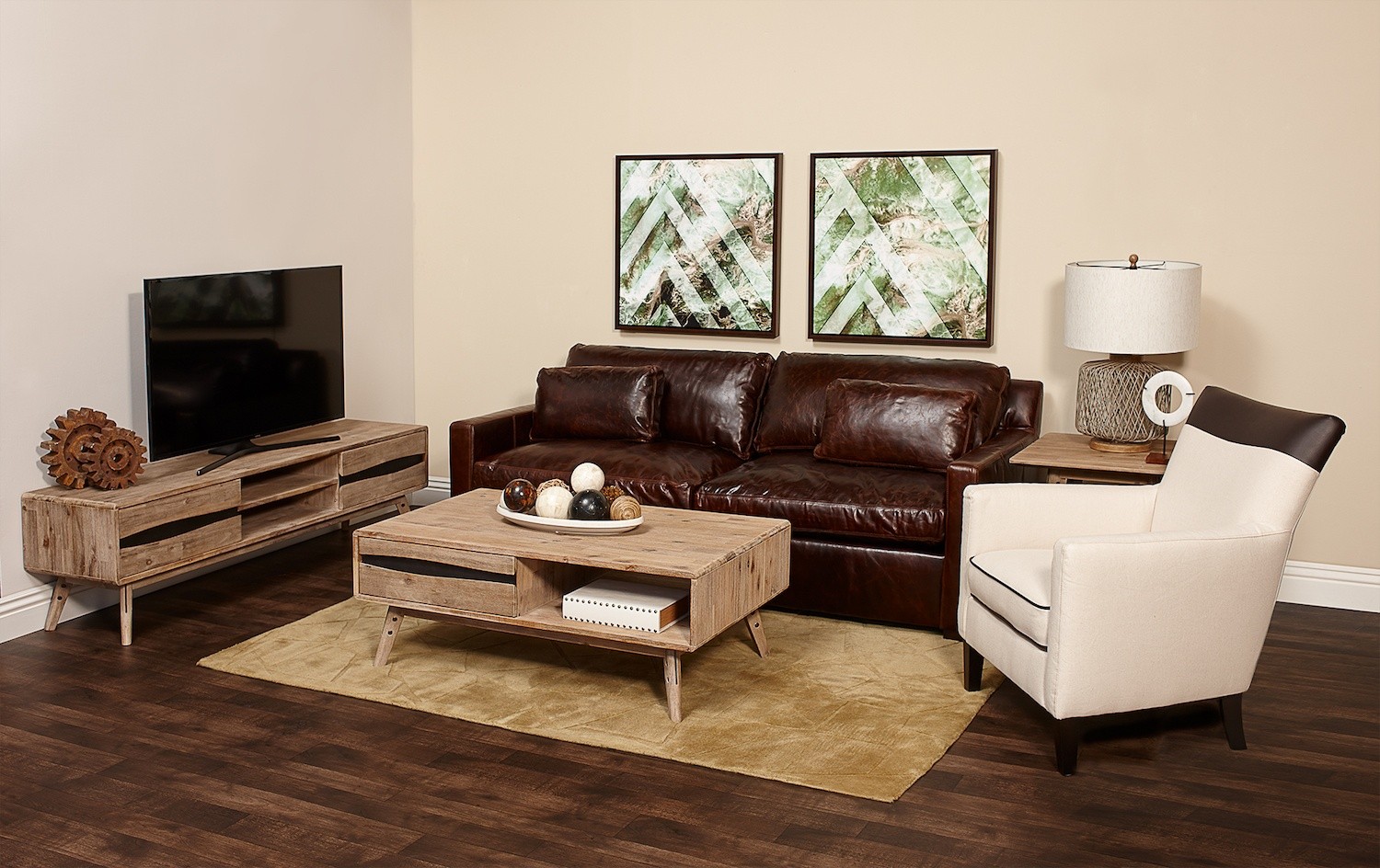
[1064,254,1203,453]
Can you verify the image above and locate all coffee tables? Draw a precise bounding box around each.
[352,487,791,723]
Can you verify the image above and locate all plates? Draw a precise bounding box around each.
[497,503,644,534]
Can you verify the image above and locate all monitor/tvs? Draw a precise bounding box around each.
[145,265,346,475]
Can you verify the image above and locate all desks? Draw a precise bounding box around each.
[1009,432,1177,486]
[20,419,429,645]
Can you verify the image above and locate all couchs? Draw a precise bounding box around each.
[958,385,1349,779]
[450,342,1048,642]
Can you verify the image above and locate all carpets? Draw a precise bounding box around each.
[196,597,1006,803]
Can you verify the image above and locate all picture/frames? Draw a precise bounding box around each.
[614,152,784,339]
[808,150,998,348]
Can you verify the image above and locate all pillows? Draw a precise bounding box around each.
[814,378,978,471]
[528,364,666,443]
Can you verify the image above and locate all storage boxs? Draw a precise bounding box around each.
[562,578,690,634]
